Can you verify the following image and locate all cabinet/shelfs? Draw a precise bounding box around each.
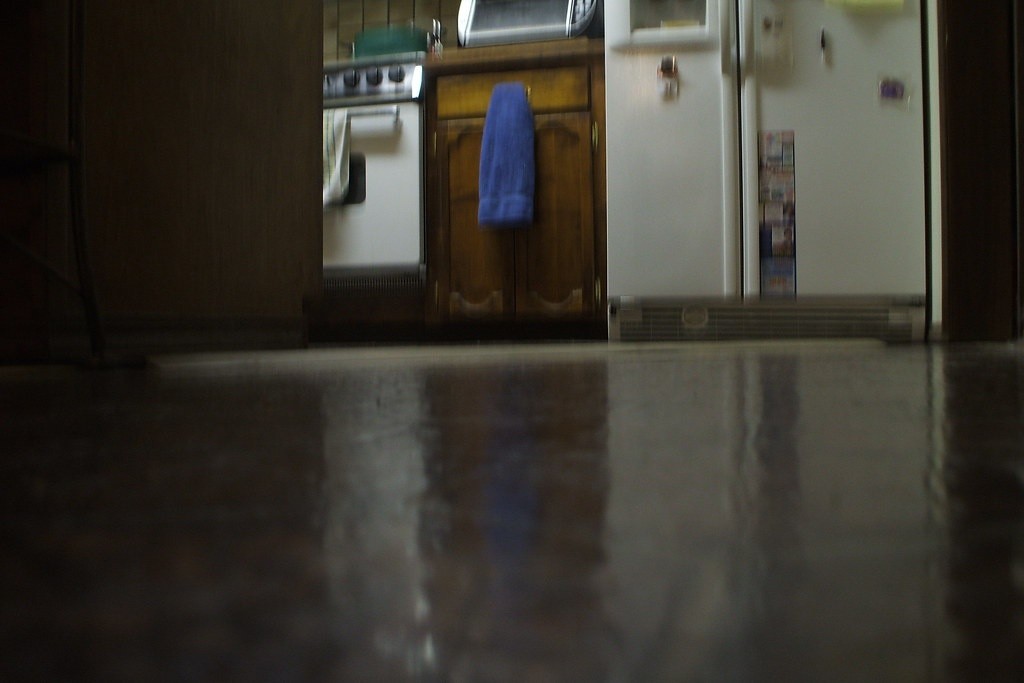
[424,37,608,342]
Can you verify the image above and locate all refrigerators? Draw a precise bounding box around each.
[603,0,927,344]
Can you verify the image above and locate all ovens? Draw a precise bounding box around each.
[320,98,426,282]
